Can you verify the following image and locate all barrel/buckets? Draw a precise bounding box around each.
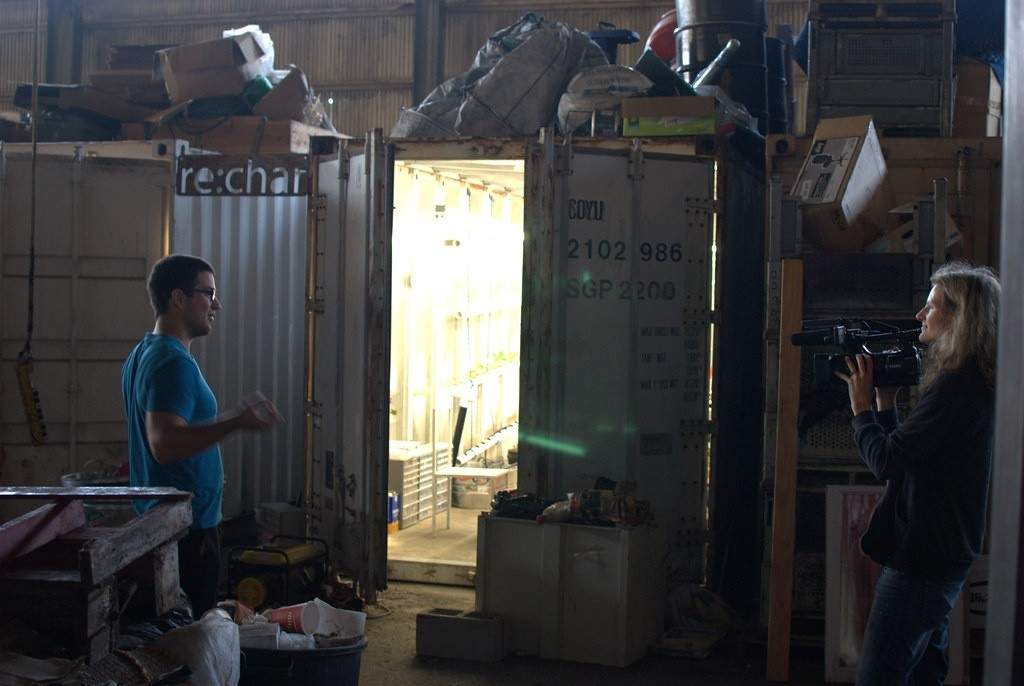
[645,10,677,62]
[673,22,769,136]
[62,471,136,528]
[675,0,768,29]
[766,37,790,135]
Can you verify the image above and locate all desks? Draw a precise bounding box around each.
[476,511,658,667]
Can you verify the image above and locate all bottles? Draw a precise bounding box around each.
[536,501,571,523]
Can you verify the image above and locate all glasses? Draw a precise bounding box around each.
[184,288,216,303]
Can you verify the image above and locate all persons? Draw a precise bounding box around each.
[835,270,1001,686]
[123,254,279,621]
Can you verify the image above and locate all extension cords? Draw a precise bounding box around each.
[16,362,50,447]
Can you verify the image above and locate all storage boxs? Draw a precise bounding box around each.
[621,96,716,136]
[790,114,900,258]
[950,65,1002,138]
[0,28,354,156]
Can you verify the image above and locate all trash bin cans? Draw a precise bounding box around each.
[474,510,662,668]
[237,632,369,686]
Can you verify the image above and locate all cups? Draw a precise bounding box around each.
[201,609,232,622]
[269,602,320,635]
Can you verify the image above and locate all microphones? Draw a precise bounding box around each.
[789,326,846,348]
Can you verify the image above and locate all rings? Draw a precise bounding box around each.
[851,370,858,376]
